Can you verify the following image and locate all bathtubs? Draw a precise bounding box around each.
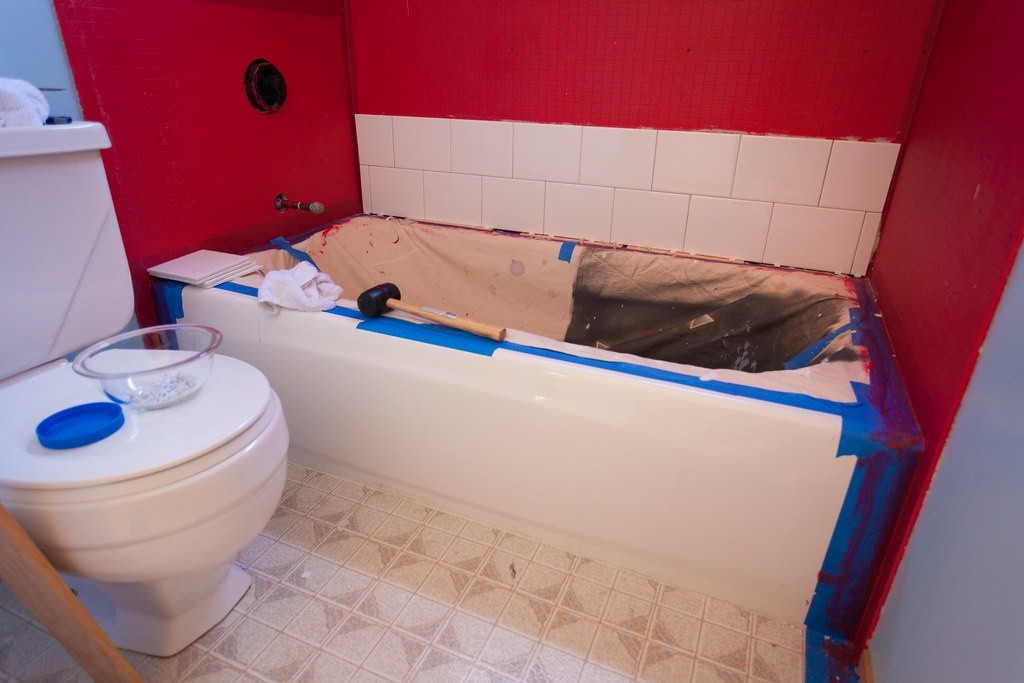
[148,213,922,637]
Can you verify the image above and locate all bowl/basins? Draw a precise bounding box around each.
[70,323,223,413]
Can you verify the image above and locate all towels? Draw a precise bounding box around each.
[257,262,342,325]
[0,78,50,126]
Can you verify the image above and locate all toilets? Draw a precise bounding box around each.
[0,121,289,656]
